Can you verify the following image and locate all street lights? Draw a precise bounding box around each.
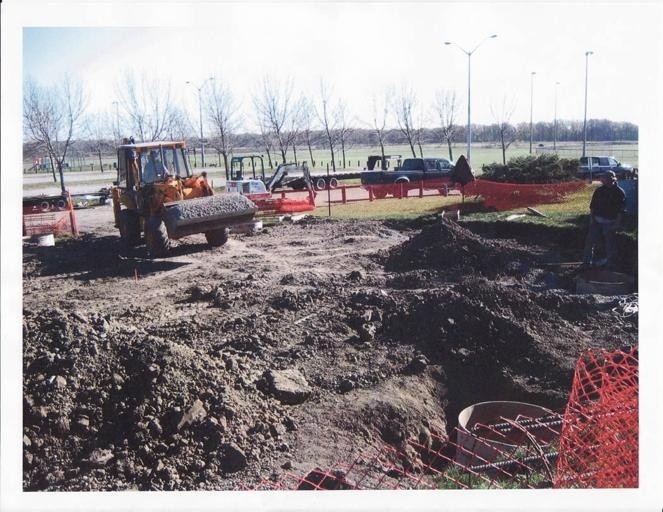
[185,78,215,166]
[527,50,594,157]
[444,34,498,162]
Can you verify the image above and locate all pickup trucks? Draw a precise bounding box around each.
[360,159,456,198]
[576,156,637,181]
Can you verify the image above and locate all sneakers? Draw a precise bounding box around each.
[575,263,591,272]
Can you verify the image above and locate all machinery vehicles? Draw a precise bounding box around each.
[226,154,318,215]
[112,136,256,259]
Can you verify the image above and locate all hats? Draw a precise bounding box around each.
[601,170,615,178]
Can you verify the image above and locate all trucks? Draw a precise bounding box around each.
[23,192,108,212]
[264,154,403,189]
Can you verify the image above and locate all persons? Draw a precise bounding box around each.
[578,170,627,273]
[143,151,169,182]
